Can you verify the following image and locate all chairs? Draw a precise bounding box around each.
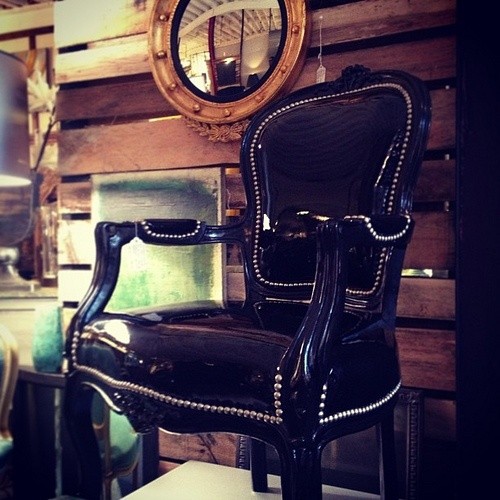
[56,68,429,497]
